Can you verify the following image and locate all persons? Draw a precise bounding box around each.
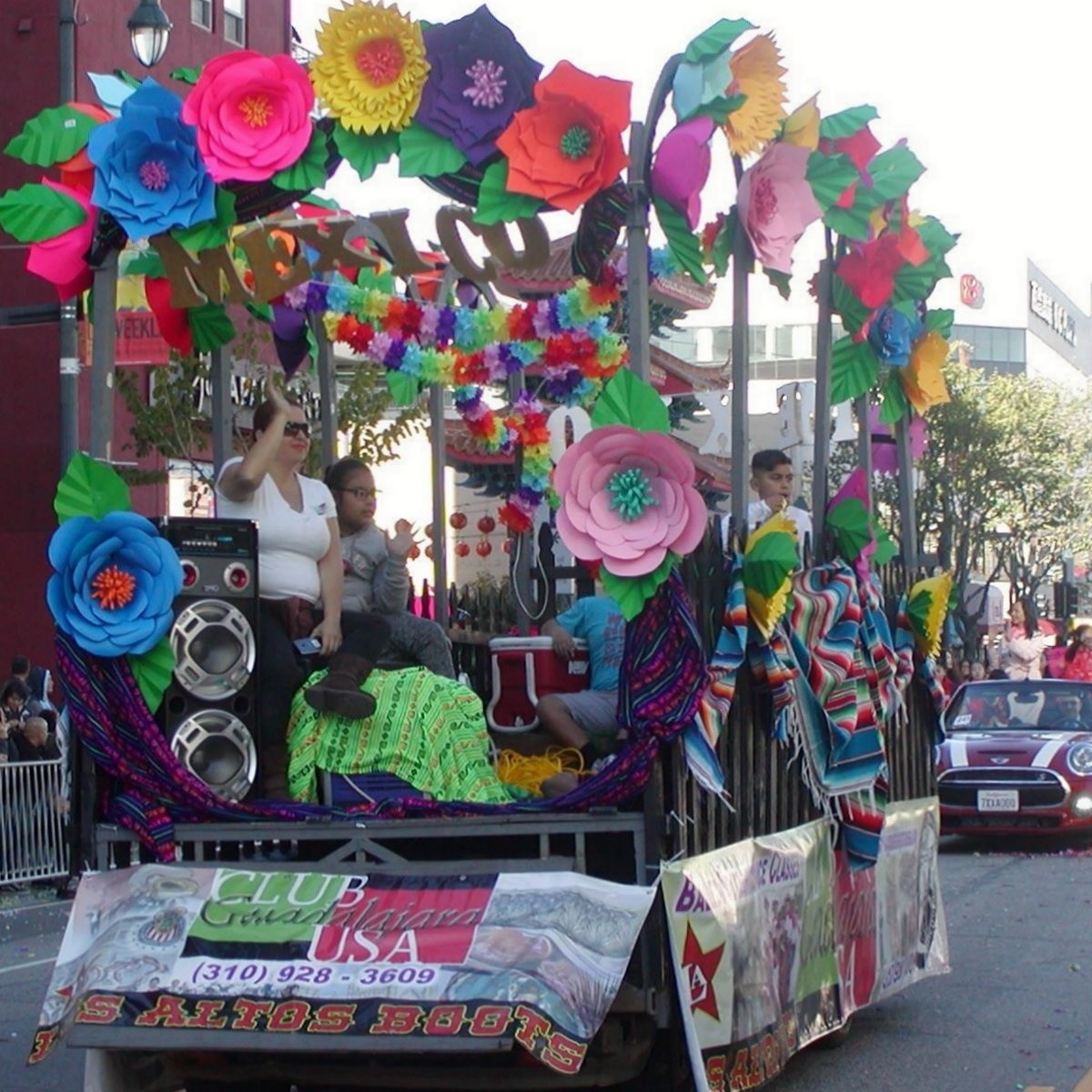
[540,595,627,769]
[321,459,455,681]
[213,371,391,717]
[999,598,1045,727]
[720,450,813,551]
[0,657,69,815]
[933,650,1009,723]
[1050,625,1092,729]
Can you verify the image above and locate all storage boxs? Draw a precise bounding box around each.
[320,770,430,807]
[484,637,591,733]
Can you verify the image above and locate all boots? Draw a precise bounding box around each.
[305,652,378,718]
[260,741,290,803]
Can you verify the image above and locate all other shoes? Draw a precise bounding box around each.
[565,754,599,771]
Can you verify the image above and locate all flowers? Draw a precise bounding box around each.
[0,0,950,721]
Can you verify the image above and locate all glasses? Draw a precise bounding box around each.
[334,488,382,500]
[283,421,313,438]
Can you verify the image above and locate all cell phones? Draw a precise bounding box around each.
[293,637,323,654]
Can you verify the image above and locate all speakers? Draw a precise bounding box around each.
[168,553,267,804]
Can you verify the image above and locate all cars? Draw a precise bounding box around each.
[933,679,1091,840]
